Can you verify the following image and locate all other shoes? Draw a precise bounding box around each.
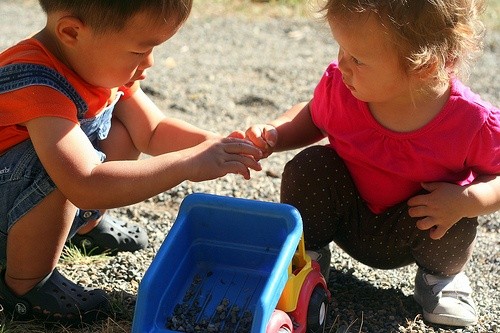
[0,265,113,328]
[65,212,149,256]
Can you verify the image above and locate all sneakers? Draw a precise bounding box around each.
[302,246,331,284]
[413,266,478,326]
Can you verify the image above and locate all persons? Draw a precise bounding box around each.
[246,1,500,327]
[0,0,263,325]
